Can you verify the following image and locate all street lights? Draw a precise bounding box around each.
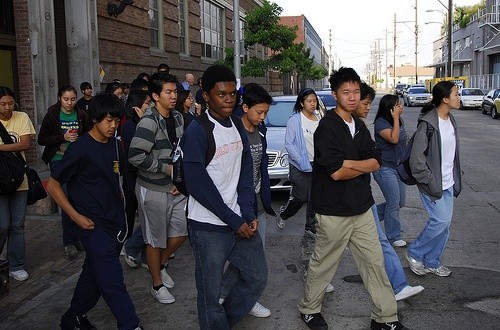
[424,9,448,77]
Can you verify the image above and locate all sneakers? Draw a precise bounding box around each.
[142,263,148,269]
[370,319,409,330]
[8,270,28,281]
[124,252,138,268]
[304,230,316,239]
[303,271,335,293]
[160,267,174,288]
[395,285,424,301]
[248,301,271,317]
[300,313,328,330]
[219,298,224,304]
[404,251,426,277]
[390,240,407,247]
[151,285,175,304]
[276,216,286,230]
[424,265,452,277]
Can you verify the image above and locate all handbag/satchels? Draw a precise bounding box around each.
[0,152,26,197]
[26,169,48,205]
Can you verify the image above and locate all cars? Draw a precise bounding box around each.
[457,87,486,110]
[264,96,327,192]
[314,90,337,110]
[482,88,500,119]
[393,83,433,107]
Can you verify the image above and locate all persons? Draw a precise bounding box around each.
[297,67,409,330]
[276,88,320,238]
[105,64,210,303]
[223,82,278,317]
[405,81,462,277]
[305,83,424,300]
[372,94,407,246]
[45,91,144,330]
[181,64,268,330]
[38,82,94,259]
[0,85,36,280]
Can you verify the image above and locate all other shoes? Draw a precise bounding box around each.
[119,324,144,330]
[169,254,174,258]
[120,245,126,255]
[60,314,97,330]
[64,245,77,256]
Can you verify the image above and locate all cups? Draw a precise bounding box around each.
[70,130,78,138]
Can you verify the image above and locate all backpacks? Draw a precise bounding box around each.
[395,121,434,185]
